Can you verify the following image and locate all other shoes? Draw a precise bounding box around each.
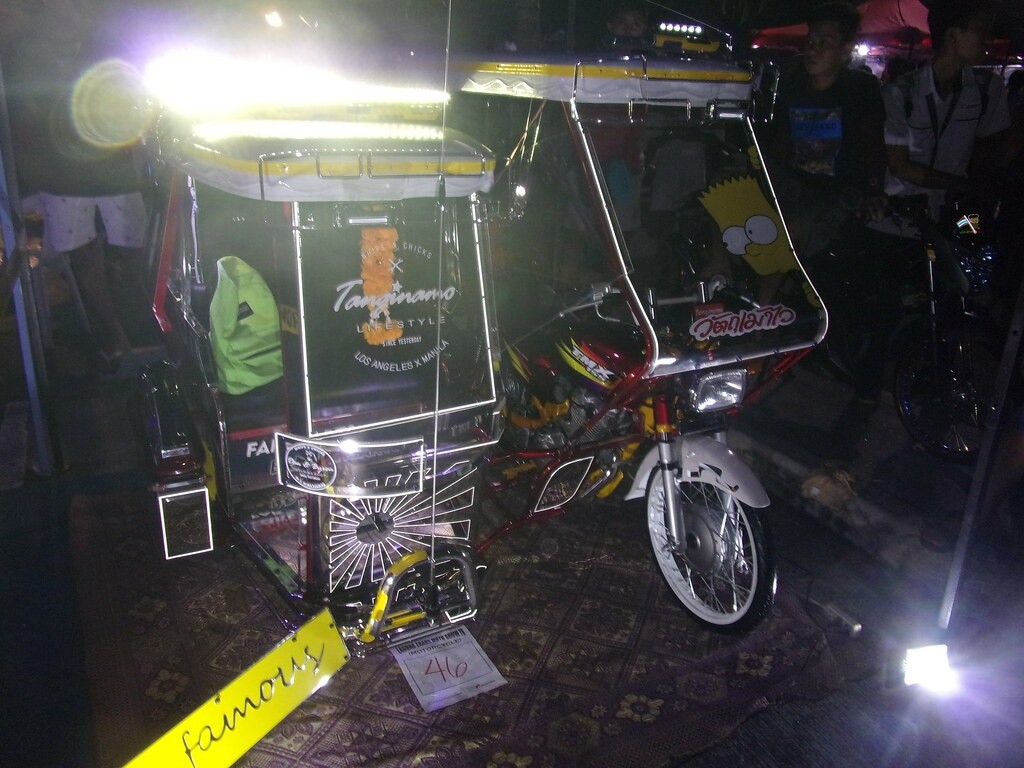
[800,463,882,510]
[757,327,801,349]
[921,503,961,552]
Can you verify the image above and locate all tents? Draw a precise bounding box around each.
[753,0,938,60]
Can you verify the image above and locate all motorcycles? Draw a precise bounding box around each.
[105,1,1024,659]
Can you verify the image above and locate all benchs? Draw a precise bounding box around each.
[220,326,427,480]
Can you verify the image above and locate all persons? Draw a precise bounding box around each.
[657,5,887,349]
[549,0,1024,298]
